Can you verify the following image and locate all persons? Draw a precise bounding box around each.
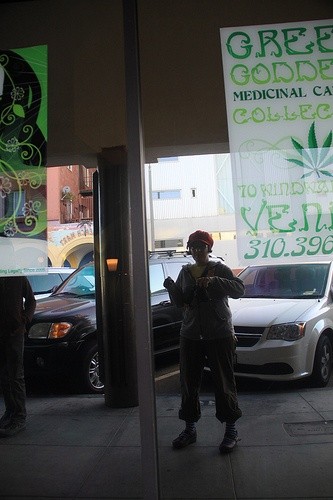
[0,276,36,438]
[163,230,246,453]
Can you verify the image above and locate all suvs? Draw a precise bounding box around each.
[24,248,226,395]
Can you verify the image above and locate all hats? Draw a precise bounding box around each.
[187,230,213,249]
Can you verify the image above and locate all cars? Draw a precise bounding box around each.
[22,267,95,304]
[203,261,333,387]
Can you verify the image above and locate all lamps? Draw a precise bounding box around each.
[106,258,120,276]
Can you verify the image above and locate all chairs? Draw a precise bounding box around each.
[270,267,318,288]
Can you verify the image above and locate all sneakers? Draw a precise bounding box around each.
[0,413,26,435]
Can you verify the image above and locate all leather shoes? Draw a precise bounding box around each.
[220,430,239,453]
[173,428,197,448]
